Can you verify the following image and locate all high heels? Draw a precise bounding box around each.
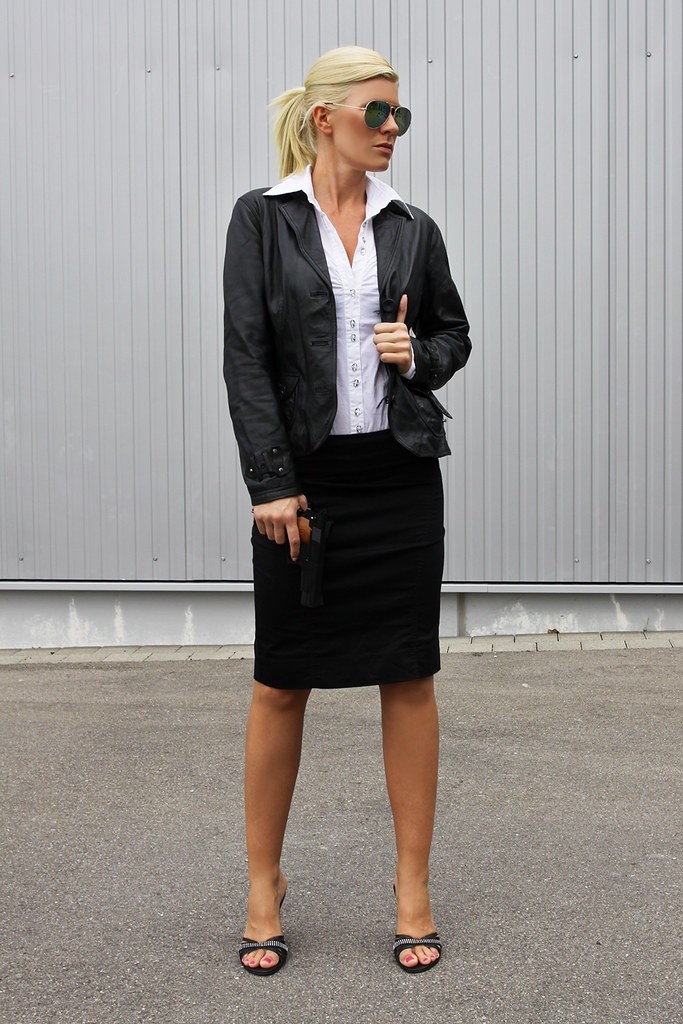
[238,877,288,975]
[392,881,442,974]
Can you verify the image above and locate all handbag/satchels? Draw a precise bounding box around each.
[377,370,453,458]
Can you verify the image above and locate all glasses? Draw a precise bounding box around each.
[325,101,411,137]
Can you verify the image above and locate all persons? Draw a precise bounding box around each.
[222,43,473,979]
